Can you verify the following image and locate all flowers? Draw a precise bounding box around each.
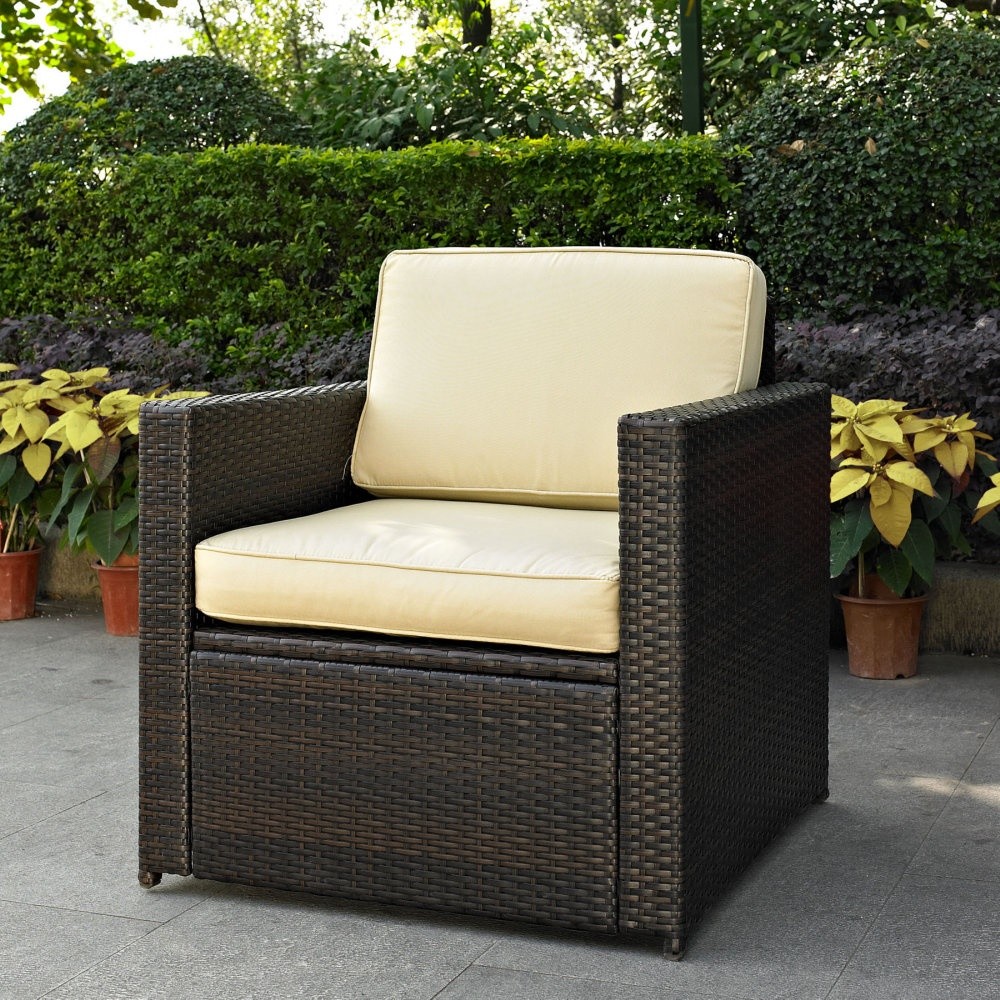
[1,362,213,566]
[832,394,1000,597]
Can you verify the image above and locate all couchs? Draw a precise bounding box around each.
[138,246,831,962]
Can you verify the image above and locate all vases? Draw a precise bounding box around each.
[0,544,46,620]
[92,558,137,636]
[836,577,924,680]
